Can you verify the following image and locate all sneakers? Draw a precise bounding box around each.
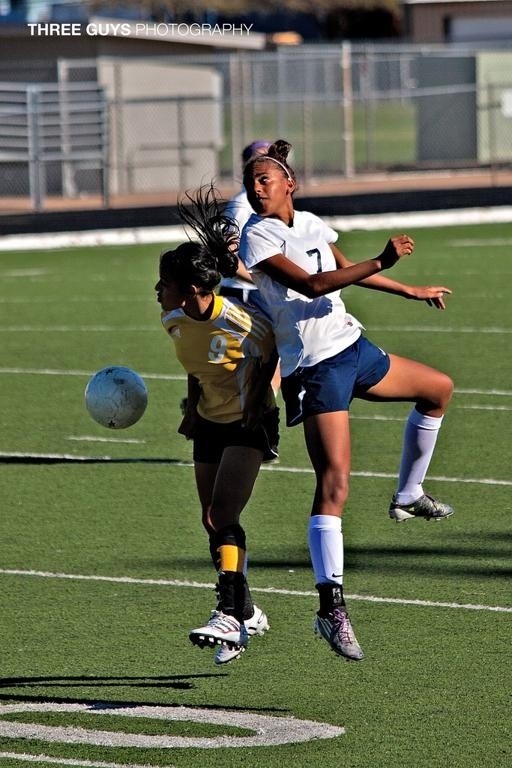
[189,613,249,649]
[314,612,365,662]
[212,606,271,665]
[388,497,453,522]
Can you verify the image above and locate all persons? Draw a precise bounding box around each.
[216,141,282,395]
[155,178,278,663]
[238,139,453,662]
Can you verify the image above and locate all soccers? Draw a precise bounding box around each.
[85,366,148,429]
